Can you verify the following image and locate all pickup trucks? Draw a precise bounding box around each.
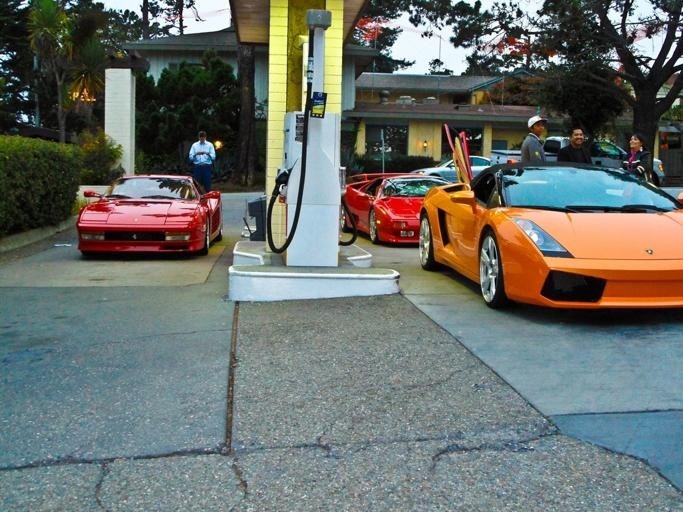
[490,136,665,185]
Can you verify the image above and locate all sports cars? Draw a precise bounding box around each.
[78,174,225,257]
[341,171,456,246]
[419,161,683,312]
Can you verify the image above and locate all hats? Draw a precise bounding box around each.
[528,116,548,127]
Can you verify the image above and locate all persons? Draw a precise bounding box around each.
[520,114,548,163]
[557,127,593,164]
[188,130,217,192]
[620,132,655,184]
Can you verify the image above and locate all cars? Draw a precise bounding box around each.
[410,155,489,183]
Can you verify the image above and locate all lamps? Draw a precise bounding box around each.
[423,140,427,148]
